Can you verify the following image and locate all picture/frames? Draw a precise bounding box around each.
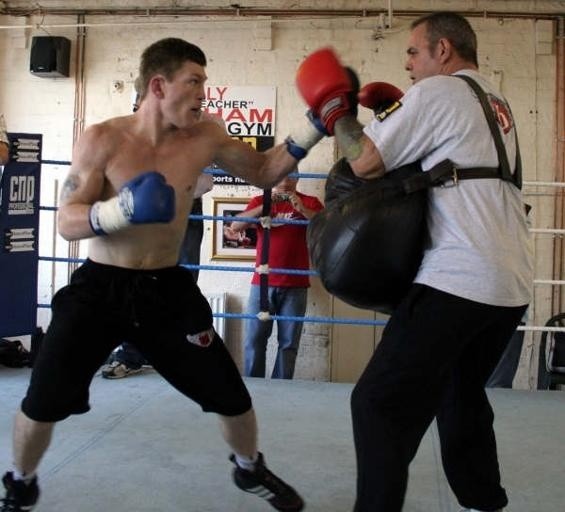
[210,198,258,262]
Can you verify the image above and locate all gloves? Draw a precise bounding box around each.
[283,50,405,160]
[88,172,175,236]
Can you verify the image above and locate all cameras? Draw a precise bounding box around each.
[278,193,289,201]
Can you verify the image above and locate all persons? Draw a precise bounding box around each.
[485,306,528,389]
[1,38,360,512]
[101,165,214,380]
[1,107,10,166]
[294,13,535,512]
[230,166,328,380]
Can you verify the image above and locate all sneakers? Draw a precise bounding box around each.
[229,453,303,512]
[102,361,144,379]
[0,472,39,512]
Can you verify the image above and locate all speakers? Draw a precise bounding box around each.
[30,35,70,78]
